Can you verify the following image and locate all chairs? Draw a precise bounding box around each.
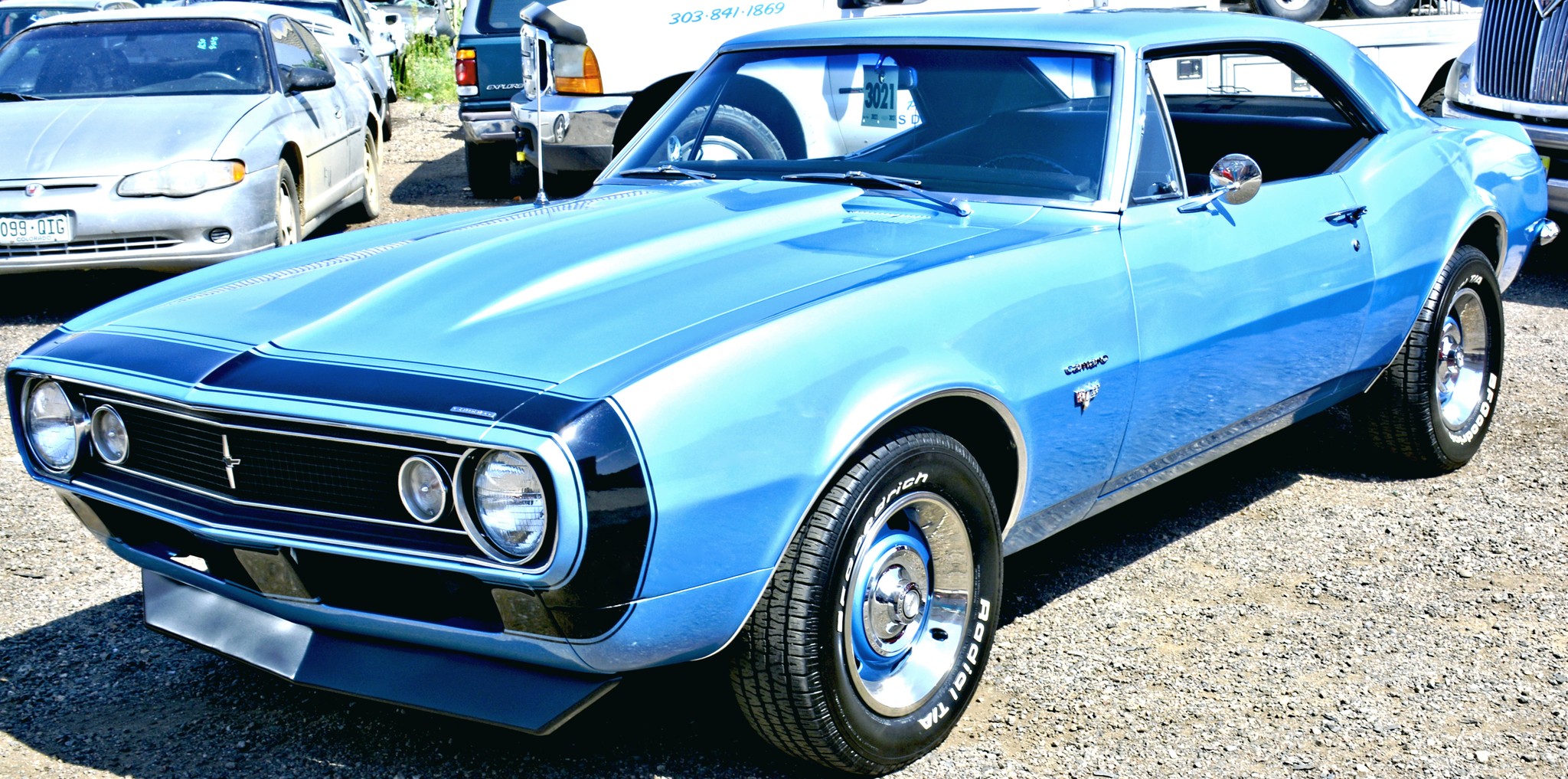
[32,42,112,96]
[216,49,266,88]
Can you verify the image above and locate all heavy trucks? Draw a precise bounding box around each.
[1438,1,1568,286]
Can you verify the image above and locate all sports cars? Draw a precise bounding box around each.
[1,1,1555,777]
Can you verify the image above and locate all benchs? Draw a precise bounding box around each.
[901,110,1352,195]
[105,48,224,91]
